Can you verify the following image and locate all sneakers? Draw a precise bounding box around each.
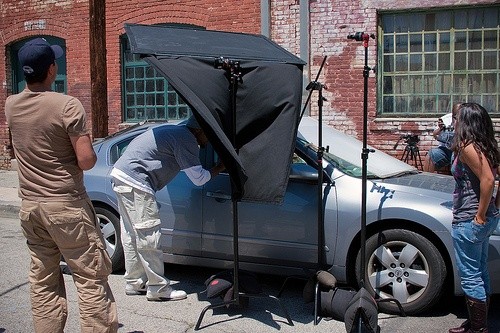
[146,285,187,301]
[125,283,147,295]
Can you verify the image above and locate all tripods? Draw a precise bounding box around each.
[401,145,423,171]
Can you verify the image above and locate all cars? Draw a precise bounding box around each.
[83,115,500,314]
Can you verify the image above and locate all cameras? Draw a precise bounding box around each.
[399,134,421,145]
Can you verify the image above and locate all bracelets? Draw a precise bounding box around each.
[474,214,487,224]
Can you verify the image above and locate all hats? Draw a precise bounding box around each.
[18,38,64,73]
[186,114,202,129]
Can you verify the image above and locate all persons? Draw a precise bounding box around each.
[5,39,117,333]
[423,104,462,173]
[448,103,500,333]
[109,114,227,301]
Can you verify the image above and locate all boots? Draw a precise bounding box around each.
[449,294,490,333]
